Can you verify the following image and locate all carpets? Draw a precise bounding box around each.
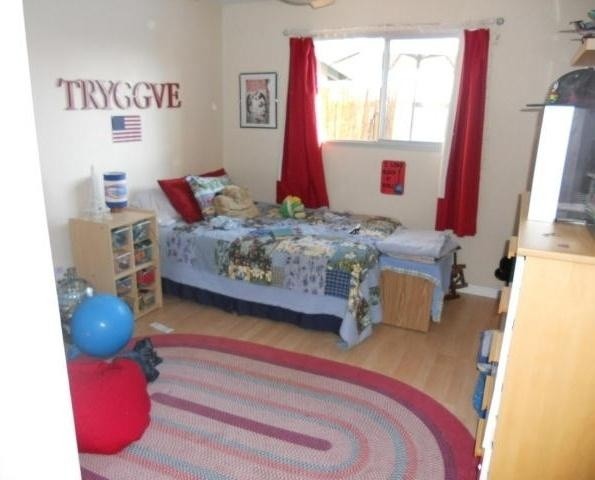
[70,332,482,479]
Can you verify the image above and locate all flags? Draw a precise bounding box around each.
[110,114,143,143]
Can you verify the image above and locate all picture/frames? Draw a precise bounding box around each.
[238,71,276,129]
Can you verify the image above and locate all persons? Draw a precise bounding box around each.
[247,91,268,125]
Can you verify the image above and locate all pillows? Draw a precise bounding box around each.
[130,187,184,226]
[186,173,236,218]
[156,166,227,224]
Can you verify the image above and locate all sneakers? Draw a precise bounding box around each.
[123,338,161,381]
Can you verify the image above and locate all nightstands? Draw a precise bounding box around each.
[69,207,164,319]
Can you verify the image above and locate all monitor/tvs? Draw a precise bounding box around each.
[526,104,595,224]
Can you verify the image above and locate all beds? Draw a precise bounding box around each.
[155,198,408,349]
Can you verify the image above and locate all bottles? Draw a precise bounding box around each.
[104,173,128,208]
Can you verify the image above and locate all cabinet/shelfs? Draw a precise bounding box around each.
[376,227,469,335]
[474,191,595,480]
